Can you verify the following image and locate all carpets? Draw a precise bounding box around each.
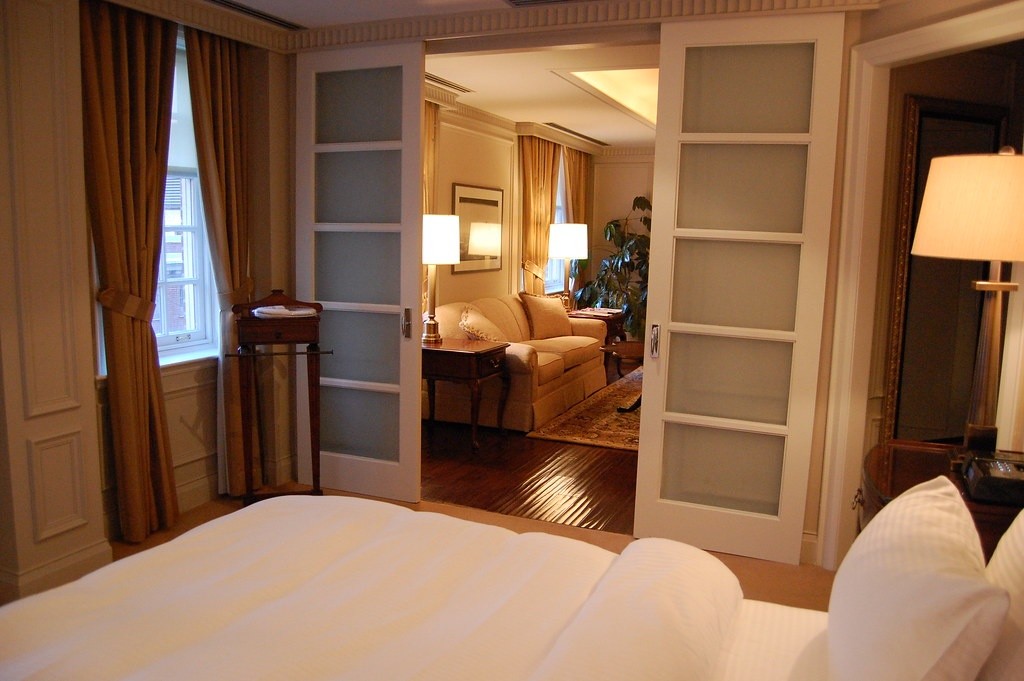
[525,364,644,451]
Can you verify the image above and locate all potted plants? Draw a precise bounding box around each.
[568,196,652,355]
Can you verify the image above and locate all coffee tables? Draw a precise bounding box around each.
[598,341,645,413]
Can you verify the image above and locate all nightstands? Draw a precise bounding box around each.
[852,438,1024,570]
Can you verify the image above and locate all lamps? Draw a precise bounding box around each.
[422,214,460,345]
[548,224,588,313]
[911,147,1023,471]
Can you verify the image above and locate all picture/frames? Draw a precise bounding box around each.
[451,182,504,275]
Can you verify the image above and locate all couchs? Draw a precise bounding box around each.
[422,290,608,434]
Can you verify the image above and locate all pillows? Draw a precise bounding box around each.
[459,304,510,347]
[984,508,1024,681]
[517,292,573,341]
[527,539,745,681]
[826,475,1015,681]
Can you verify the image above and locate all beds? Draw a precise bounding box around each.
[0,493,1024,681]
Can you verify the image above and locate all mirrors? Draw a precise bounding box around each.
[876,91,1013,447]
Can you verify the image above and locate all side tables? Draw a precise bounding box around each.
[566,307,627,377]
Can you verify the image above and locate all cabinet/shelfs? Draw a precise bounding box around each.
[224,288,333,507]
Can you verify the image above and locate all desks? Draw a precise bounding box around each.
[421,336,512,452]
